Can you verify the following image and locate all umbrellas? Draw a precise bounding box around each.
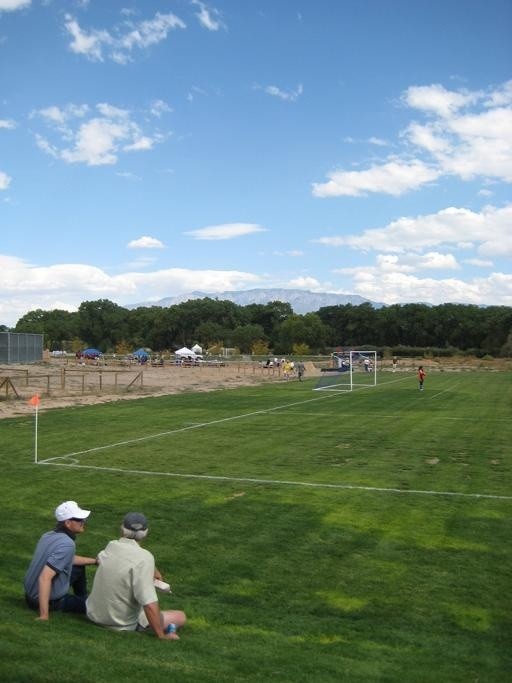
[83,349,102,354]
[175,344,203,355]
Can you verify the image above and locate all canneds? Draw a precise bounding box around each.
[167,623,176,634]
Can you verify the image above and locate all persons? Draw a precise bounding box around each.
[268,358,307,380]
[391,355,399,372]
[417,366,427,390]
[83,510,187,641]
[334,354,373,372]
[22,501,99,623]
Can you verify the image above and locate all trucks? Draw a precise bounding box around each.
[176,353,203,361]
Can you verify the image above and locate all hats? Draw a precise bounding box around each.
[55,500,91,522]
[124,512,148,531]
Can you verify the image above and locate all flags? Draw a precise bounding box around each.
[28,394,39,406]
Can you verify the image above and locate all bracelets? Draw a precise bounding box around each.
[94,558,98,564]
